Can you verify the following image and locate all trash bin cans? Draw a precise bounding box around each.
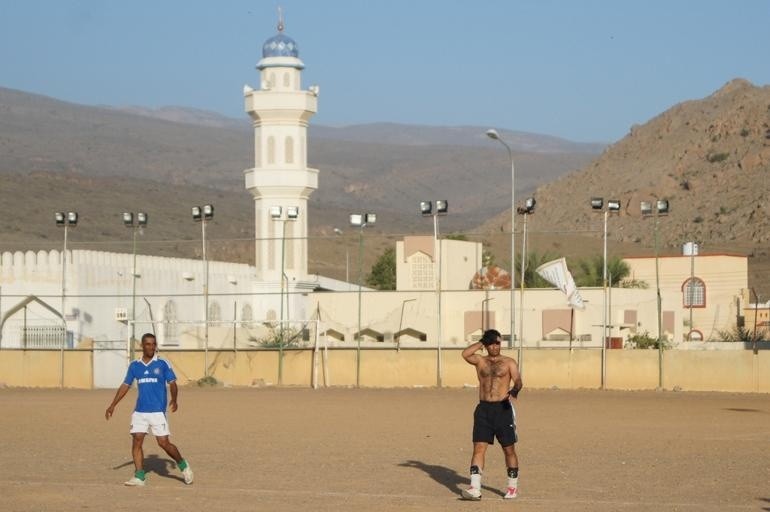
[605,336,623,350]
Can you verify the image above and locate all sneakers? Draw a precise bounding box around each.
[505,487,518,499]
[124,477,145,487]
[182,460,193,484]
[463,487,481,499]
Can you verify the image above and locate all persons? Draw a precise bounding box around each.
[458,327,524,501]
[105,332,194,485]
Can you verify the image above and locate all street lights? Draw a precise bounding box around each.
[348,211,379,388]
[192,204,214,389]
[123,212,148,389]
[267,205,299,385]
[517,197,537,390]
[486,128,518,348]
[53,211,78,389]
[591,196,621,388]
[640,198,669,387]
[421,199,449,387]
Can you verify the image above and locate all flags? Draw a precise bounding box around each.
[534,255,588,315]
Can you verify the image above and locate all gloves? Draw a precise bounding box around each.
[480,331,496,346]
[508,389,519,399]
